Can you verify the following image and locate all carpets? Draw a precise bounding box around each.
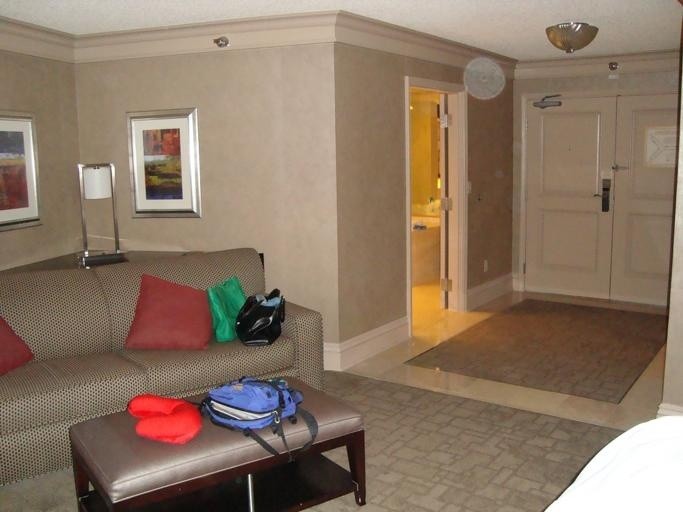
[0,369,626,512]
[402,298,667,404]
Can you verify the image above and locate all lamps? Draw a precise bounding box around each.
[544,22,598,53]
[75,161,129,270]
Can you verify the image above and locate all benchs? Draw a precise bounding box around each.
[67,376,367,512]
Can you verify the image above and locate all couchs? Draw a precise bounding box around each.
[0,247,325,487]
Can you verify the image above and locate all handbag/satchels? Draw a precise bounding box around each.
[207,276,246,343]
[199,376,317,464]
[235,288,284,347]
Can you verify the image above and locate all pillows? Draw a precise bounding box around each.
[0,314,33,381]
[123,273,213,352]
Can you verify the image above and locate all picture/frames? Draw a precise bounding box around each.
[125,106,204,218]
[0,109,43,232]
